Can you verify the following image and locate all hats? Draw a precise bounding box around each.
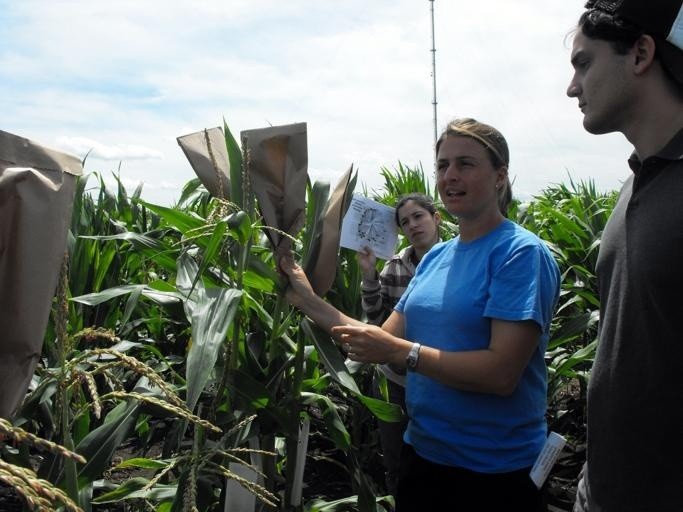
[584,1,683,50]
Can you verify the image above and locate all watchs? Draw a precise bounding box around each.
[406,342,420,372]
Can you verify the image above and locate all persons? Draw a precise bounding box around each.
[566,0,682,511]
[356,191,446,495]
[279,116,562,512]
